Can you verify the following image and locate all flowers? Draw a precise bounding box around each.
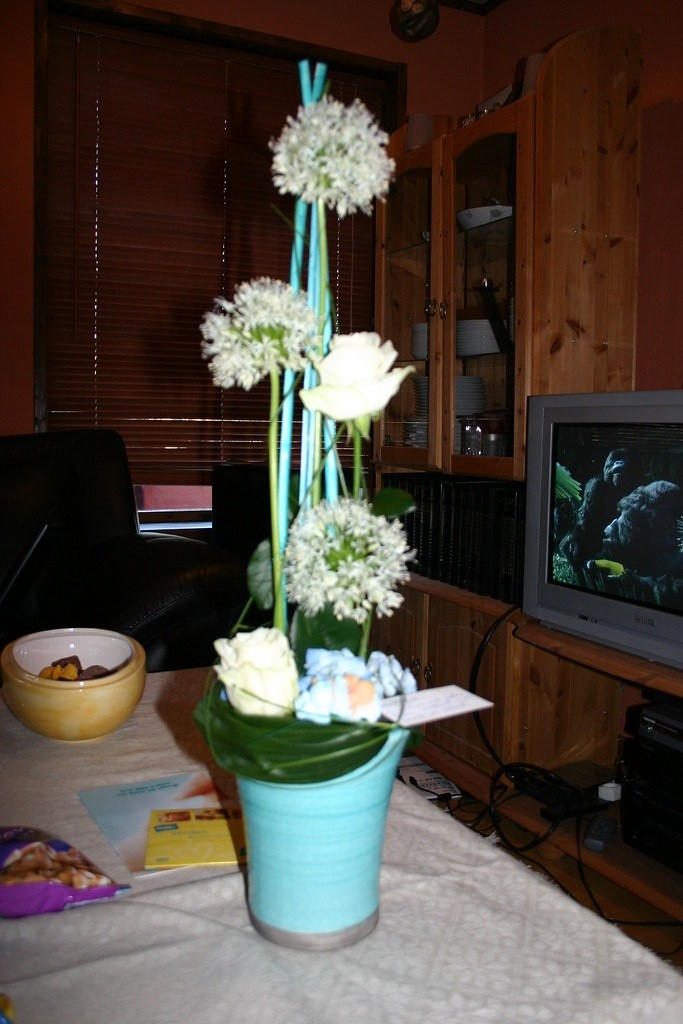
[195,61,421,782]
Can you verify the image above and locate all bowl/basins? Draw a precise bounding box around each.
[0,627,146,743]
[456,206,513,248]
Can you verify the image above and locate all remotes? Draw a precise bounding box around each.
[540,798,609,823]
[584,814,617,851]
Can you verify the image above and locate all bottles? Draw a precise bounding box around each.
[484,422,506,457]
[460,420,483,455]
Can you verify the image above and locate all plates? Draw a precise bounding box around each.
[410,318,499,361]
[402,375,489,455]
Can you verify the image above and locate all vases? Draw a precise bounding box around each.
[236,654,418,954]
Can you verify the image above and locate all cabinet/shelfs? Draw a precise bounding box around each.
[367,28,683,922]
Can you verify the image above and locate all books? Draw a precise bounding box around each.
[144,807,247,871]
[398,756,463,800]
[77,771,237,879]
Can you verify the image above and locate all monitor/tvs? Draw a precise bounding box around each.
[521,389,683,671]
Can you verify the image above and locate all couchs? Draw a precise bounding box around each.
[0,429,253,673]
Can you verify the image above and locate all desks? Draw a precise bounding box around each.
[0,666,683,1024]
